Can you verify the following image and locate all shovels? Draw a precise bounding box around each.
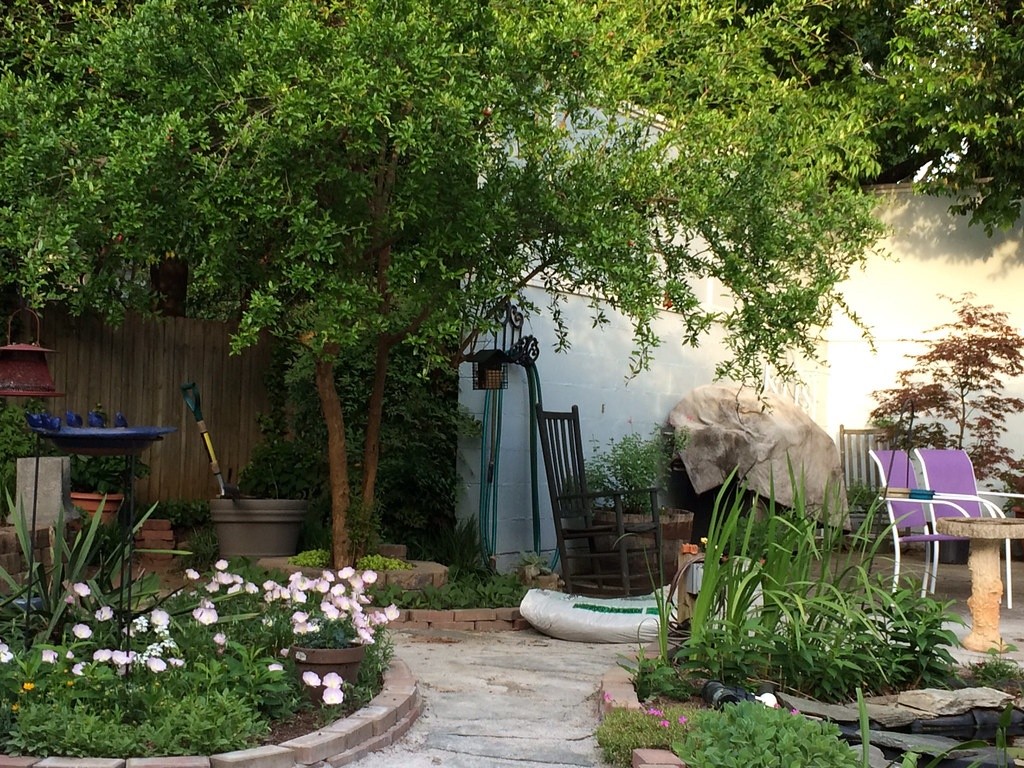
[179,382,229,499]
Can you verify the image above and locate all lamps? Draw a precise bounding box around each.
[467,334,563,573]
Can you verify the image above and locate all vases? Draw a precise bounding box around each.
[291,643,366,704]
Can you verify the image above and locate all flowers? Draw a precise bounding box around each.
[285,584,378,649]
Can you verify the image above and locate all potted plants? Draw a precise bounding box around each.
[867,292,1024,565]
[846,480,891,554]
[581,422,695,596]
[69,453,154,530]
[209,440,309,557]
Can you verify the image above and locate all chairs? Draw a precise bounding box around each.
[868,448,1024,610]
[534,401,668,597]
